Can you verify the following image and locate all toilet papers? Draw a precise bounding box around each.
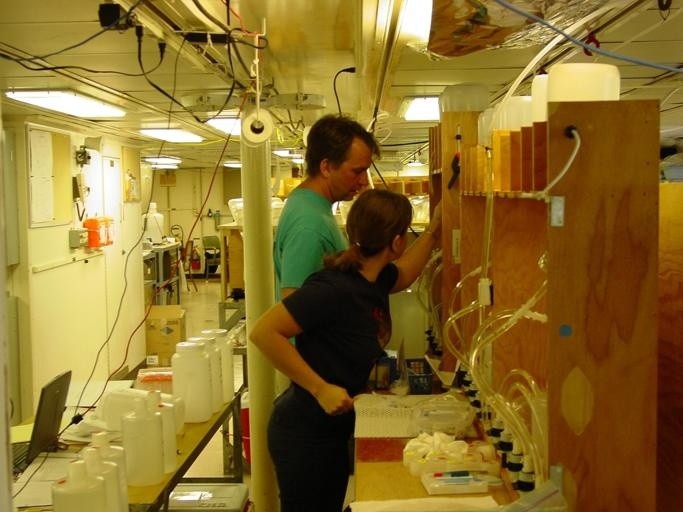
[241,109,274,148]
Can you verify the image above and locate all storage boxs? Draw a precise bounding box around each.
[143,303,190,368]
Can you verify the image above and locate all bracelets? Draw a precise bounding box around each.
[424,227,437,240]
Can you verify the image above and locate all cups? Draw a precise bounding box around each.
[476,61,620,196]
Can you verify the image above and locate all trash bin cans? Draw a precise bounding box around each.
[145,305,185,366]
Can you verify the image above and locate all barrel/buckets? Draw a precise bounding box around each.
[50,386,187,512]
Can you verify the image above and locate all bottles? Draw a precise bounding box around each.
[120,398,165,489]
[170,329,234,423]
[374,356,390,390]
[145,389,178,474]
[49,430,131,511]
[96,387,186,438]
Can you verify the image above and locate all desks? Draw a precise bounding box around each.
[6,372,250,512]
[352,356,517,512]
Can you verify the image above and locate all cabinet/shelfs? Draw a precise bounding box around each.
[427,94,682,512]
[152,240,183,306]
[141,251,159,310]
[213,218,281,357]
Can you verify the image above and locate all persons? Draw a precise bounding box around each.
[272,115,383,398]
[249,188,445,511]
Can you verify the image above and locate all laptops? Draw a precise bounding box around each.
[11,370,71,473]
